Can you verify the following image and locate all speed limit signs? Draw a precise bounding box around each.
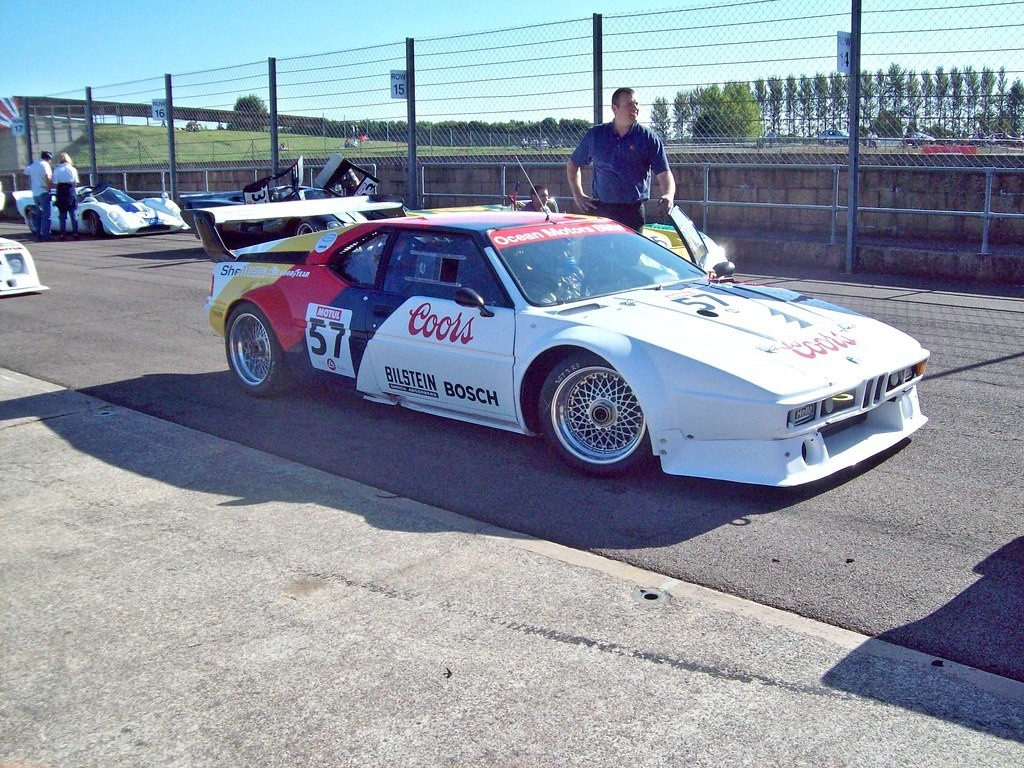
[12,116,25,136]
[390,69,408,100]
[151,99,168,121]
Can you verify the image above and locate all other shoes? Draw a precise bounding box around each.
[73,234,80,240]
[59,236,67,241]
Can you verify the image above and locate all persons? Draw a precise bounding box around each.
[218,124,225,129]
[340,169,360,189]
[360,134,368,142]
[521,184,551,213]
[872,132,879,148]
[567,87,675,264]
[24,150,52,236]
[345,139,357,148]
[161,120,166,128]
[187,124,201,132]
[279,143,286,150]
[52,152,80,237]
[541,137,548,146]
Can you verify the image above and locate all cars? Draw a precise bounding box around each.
[401,203,731,277]
[12,182,191,235]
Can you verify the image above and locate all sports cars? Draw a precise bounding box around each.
[207,205,931,493]
